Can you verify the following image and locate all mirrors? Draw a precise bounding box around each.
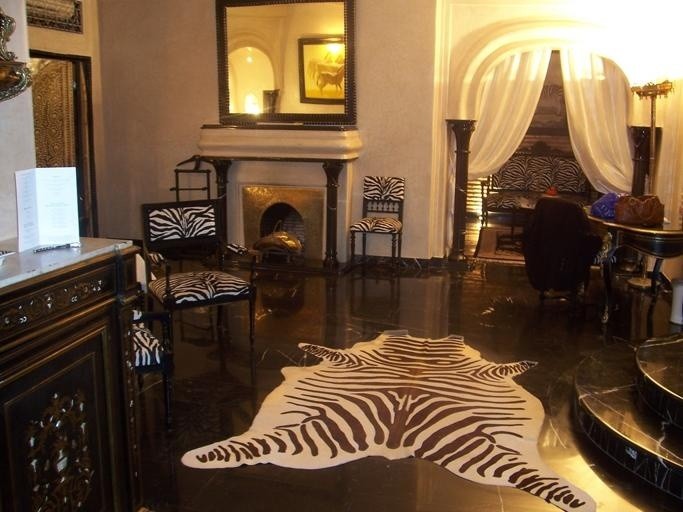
[216,0,356,124]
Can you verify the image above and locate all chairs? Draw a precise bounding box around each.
[350,176,405,277]
[133,199,260,429]
[523,197,604,310]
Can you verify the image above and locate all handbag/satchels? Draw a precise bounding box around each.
[614,196,665,227]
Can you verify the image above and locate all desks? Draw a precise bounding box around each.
[583,206,683,296]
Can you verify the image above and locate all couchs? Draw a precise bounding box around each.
[481,141,592,244]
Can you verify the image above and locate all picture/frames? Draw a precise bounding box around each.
[298,36,346,104]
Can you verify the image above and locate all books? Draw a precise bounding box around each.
[13,166,79,254]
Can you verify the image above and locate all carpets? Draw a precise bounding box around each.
[181,328,598,512]
[473,227,525,268]
